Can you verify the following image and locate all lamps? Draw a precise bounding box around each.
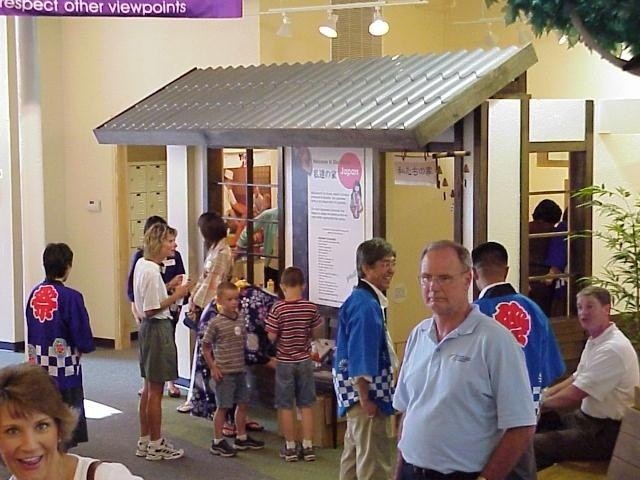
[367,5,390,38]
[317,6,339,39]
[275,11,293,39]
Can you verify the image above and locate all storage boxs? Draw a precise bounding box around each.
[278,392,335,448]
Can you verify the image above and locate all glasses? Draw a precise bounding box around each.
[418,269,470,288]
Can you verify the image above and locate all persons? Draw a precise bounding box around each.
[533,286,640,471]
[471,242,567,480]
[544,207,568,319]
[392,239,538,480]
[26,243,94,454]
[0,362,145,480]
[265,266,326,461]
[332,237,399,480]
[529,199,562,318]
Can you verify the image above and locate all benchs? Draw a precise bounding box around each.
[535,405,640,480]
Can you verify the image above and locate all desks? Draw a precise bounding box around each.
[314,371,347,446]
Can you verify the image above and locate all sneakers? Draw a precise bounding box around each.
[208,440,236,457]
[233,435,265,450]
[279,446,300,462]
[147,440,184,462]
[135,440,148,457]
[301,445,317,462]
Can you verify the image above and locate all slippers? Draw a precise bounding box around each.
[243,419,265,432]
[138,383,182,398]
[220,423,238,438]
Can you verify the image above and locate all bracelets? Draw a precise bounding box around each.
[476,476,487,480]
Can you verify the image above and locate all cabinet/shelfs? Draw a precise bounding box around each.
[127,160,167,334]
